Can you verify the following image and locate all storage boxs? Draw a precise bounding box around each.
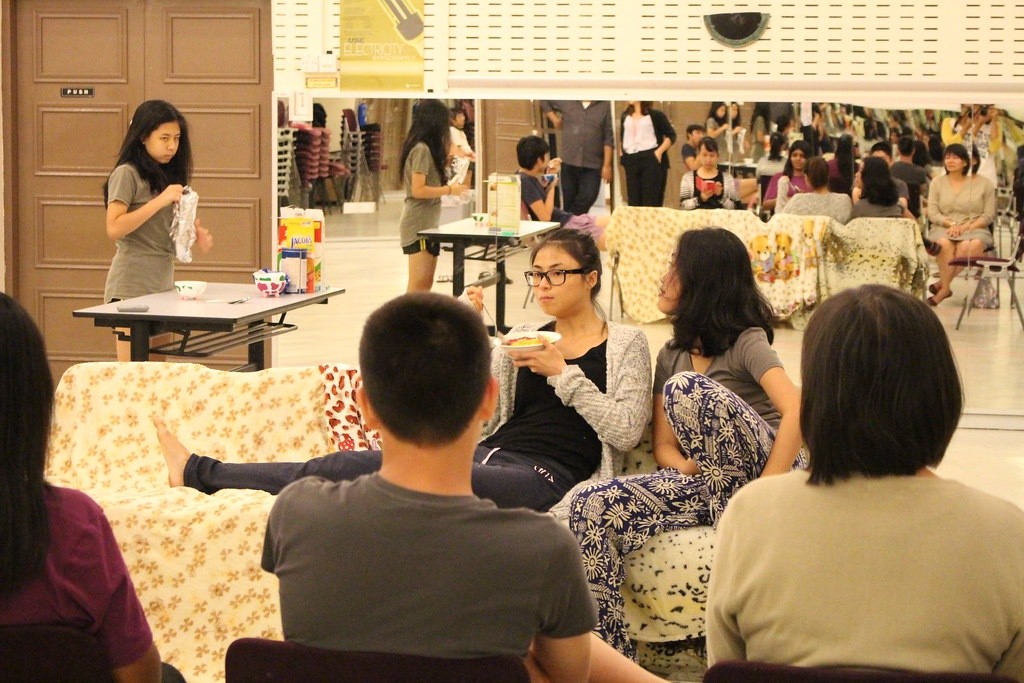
[280,216,315,250]
[307,255,323,292]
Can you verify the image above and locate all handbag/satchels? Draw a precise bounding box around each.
[278,206,325,291]
[168,185,198,263]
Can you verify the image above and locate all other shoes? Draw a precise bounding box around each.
[929,284,937,295]
[928,291,952,306]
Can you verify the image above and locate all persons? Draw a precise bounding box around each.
[0,292,164,683]
[563,225,804,661]
[514,137,603,248]
[680,129,998,305]
[102,98,213,364]
[399,99,470,291]
[442,108,477,207]
[707,283,1024,683]
[149,225,652,515]
[263,288,671,683]
[538,100,614,213]
[708,100,1024,197]
[618,100,677,209]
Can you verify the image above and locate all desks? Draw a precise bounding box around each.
[70,280,346,373]
[416,216,562,337]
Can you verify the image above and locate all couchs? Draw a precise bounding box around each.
[49,361,716,683]
[600,207,930,331]
[264,84,1024,418]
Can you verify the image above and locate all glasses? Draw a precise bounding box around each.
[524,267,585,287]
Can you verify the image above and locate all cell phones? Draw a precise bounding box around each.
[705,180,716,192]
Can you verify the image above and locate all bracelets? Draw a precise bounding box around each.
[444,184,452,196]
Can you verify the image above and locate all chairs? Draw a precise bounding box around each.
[990,184,1015,256]
[225,638,531,683]
[277,99,388,220]
[946,219,1024,331]
[703,661,1020,683]
[903,180,924,220]
[0,620,115,683]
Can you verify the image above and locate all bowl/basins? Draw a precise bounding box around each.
[471,212,488,224]
[174,281,207,301]
[540,174,558,184]
[493,331,562,360]
[253,272,287,298]
